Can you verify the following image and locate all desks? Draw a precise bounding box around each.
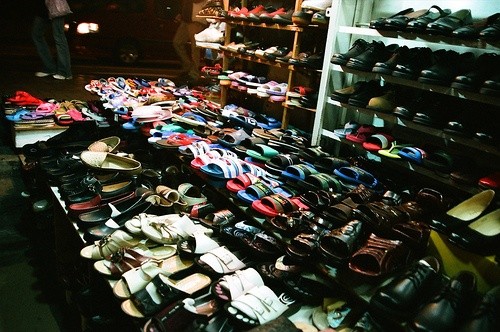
[15,119,109,147]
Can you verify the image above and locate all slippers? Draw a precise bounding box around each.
[194,1,328,83]
[5,80,500,332]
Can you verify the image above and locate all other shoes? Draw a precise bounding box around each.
[53,74,73,80]
[35,71,56,77]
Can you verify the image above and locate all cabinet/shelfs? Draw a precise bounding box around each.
[33,0,500,332]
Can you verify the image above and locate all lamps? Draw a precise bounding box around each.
[70,18,90,34]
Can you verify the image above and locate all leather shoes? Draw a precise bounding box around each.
[480,13,500,37]
[426,9,473,34]
[372,45,418,74]
[369,8,414,29]
[385,9,428,28]
[347,40,399,72]
[452,52,497,91]
[480,55,500,94]
[330,39,372,65]
[419,49,460,83]
[393,47,433,77]
[407,5,451,31]
[452,24,486,39]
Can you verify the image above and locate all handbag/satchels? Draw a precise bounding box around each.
[45,0,73,19]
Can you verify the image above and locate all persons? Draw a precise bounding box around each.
[171,0,210,81]
[31,0,73,79]
